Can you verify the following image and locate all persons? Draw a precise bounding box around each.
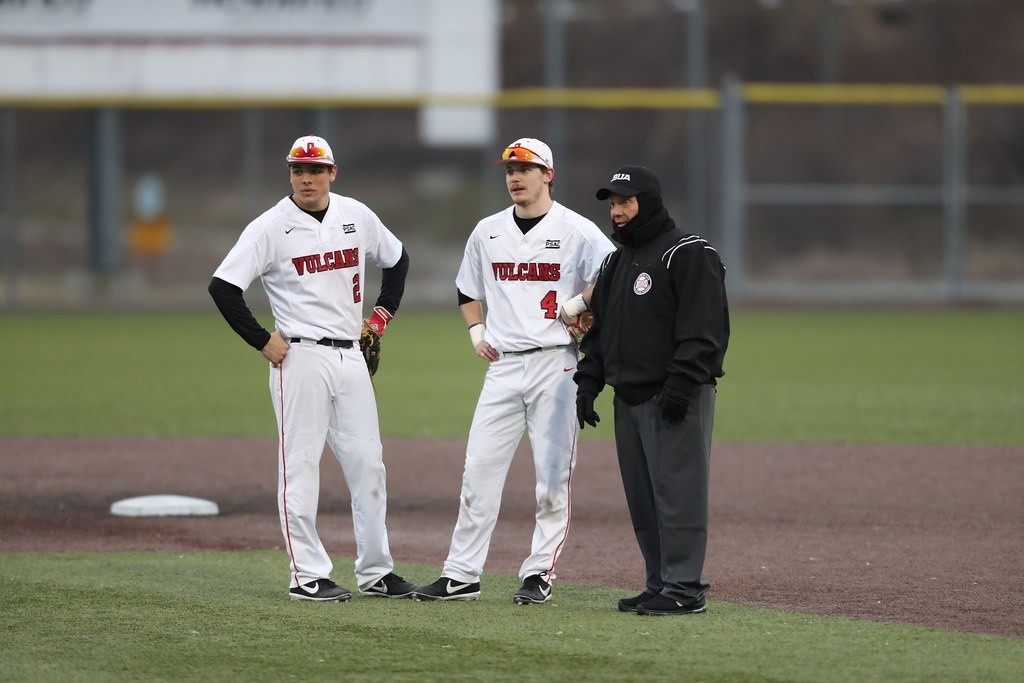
[573,166,735,620]
[205,124,416,603]
[410,135,619,609]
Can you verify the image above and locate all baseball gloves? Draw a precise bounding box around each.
[359,317,383,377]
[566,310,593,343]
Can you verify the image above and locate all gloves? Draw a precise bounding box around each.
[367,306,394,339]
[653,386,689,431]
[575,392,601,429]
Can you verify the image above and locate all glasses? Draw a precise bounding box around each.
[501,146,549,167]
[288,146,328,159]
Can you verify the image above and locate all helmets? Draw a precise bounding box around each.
[285,133,336,166]
[492,138,555,170]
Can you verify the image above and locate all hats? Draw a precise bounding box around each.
[595,166,662,201]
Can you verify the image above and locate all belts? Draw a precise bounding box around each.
[290,337,363,349]
[503,344,568,354]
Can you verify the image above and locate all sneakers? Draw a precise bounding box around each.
[361,572,418,599]
[288,579,353,602]
[412,578,481,601]
[513,574,553,604]
[617,591,708,616]
